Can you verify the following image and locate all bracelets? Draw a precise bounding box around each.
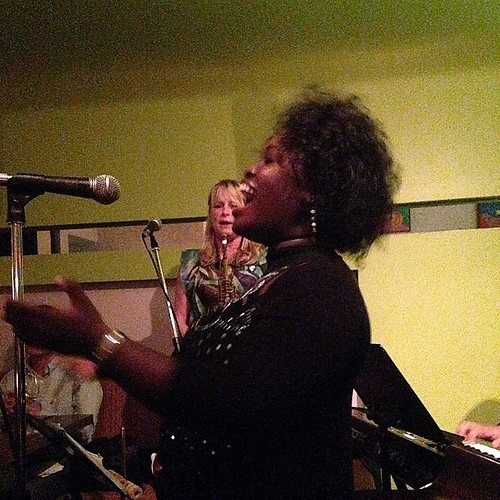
[89,327,128,366]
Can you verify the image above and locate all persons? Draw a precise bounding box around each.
[0,305,104,446]
[455,419,500,449]
[173,179,267,338]
[2,89,402,500]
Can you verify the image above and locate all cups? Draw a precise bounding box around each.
[24,369,39,396]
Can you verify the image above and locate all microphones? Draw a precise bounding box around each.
[142,218,162,237]
[0,172,121,206]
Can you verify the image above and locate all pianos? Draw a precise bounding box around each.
[353,405,500,500]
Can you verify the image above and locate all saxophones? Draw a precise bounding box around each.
[217,233,239,308]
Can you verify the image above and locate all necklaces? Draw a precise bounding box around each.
[240,259,295,298]
